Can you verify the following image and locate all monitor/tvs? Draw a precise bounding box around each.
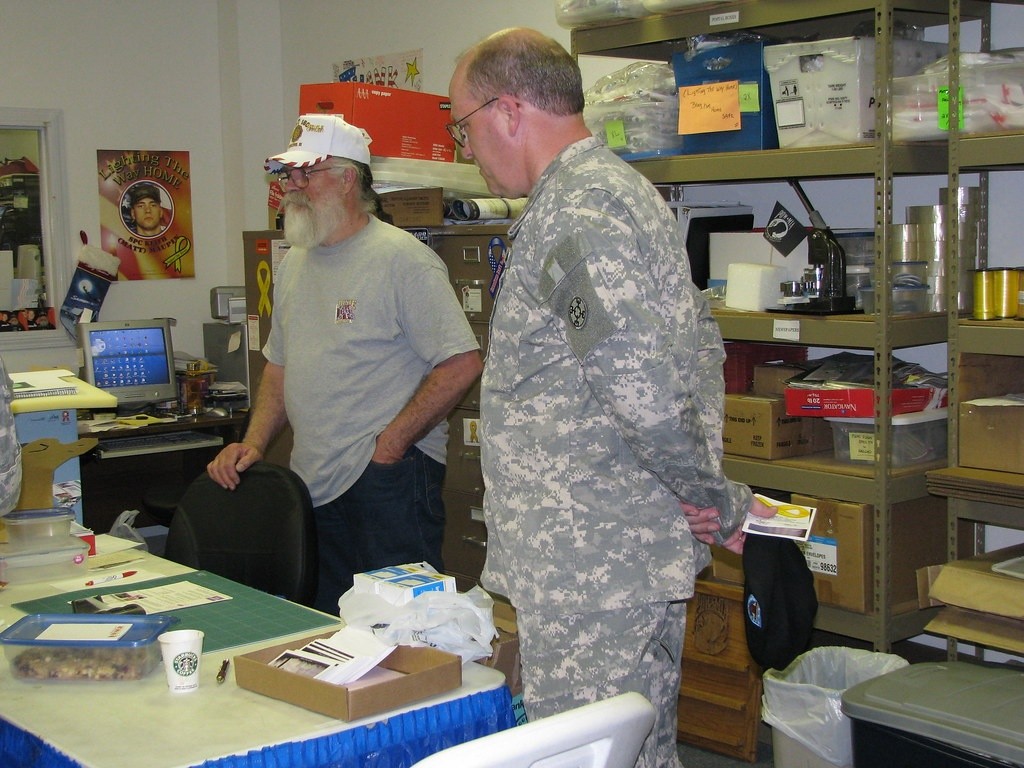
[75,319,177,417]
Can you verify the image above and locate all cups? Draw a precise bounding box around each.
[157,629,205,695]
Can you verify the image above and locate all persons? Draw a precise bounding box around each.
[207,113,483,618]
[128,183,162,236]
[446,26,781,767]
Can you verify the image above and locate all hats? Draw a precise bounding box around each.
[742,533,818,670]
[130,186,161,207]
[264,115,370,175]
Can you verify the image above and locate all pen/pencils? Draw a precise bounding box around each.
[84,571,138,586]
[216,659,230,683]
[177,414,196,418]
[116,415,148,421]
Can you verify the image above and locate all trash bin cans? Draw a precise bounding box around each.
[761,646,910,768]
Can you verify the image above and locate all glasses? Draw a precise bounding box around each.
[446,98,522,149]
[277,166,340,192]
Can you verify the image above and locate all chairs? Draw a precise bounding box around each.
[414,692,654,768]
[167,460,320,604]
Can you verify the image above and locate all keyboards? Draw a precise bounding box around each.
[94,431,224,458]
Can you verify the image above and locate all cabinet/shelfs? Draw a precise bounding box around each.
[571,2,994,659]
[941,3,1023,668]
[241,227,513,583]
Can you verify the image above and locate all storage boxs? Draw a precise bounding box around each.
[371,190,444,226]
[474,602,523,698]
[229,629,460,720]
[299,83,457,166]
[672,35,1024,766]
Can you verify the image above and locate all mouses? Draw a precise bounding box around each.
[205,408,229,418]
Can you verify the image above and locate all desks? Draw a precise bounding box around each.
[77,406,245,540]
[1,522,517,767]
